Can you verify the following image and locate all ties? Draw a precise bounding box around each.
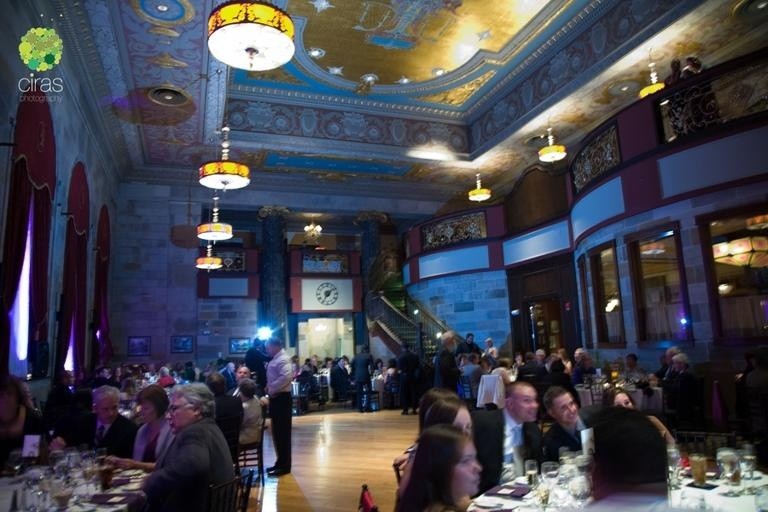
[98,425,105,439]
[574,429,581,443]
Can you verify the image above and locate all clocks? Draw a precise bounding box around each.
[316,282,338,305]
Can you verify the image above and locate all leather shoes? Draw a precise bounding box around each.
[266,466,276,471]
[269,469,289,476]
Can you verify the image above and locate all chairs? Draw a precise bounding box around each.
[393,462,404,484]
[358,484,379,511]
[461,368,581,436]
[590,381,615,405]
[290,375,401,416]
[207,468,254,512]
[235,403,267,486]
[671,373,768,439]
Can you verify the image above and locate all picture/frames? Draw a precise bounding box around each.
[229,338,250,354]
[128,336,151,356]
[170,335,193,353]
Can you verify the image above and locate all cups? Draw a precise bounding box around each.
[9,446,108,512]
[577,363,659,389]
[525,444,593,510]
[667,444,766,512]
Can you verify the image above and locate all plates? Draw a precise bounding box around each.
[63,466,150,511]
[475,473,545,511]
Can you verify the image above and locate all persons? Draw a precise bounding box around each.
[683,53,720,127]
[665,59,694,140]
[393,378,680,510]
[436,328,768,468]
[1,337,294,510]
[305,343,419,416]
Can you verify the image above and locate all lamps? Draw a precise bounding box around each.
[468,172,491,203]
[638,61,665,98]
[206,1,296,73]
[303,215,323,243]
[712,237,768,269]
[194,65,252,275]
[538,127,567,163]
